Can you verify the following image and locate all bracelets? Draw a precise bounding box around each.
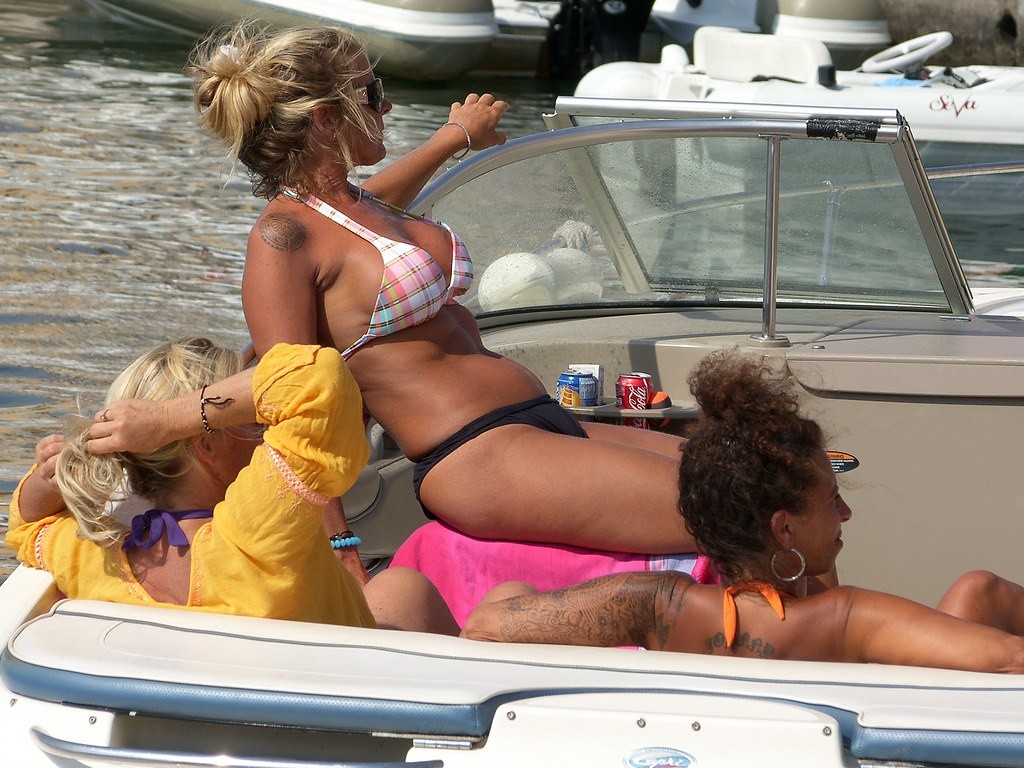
[443,123,471,160]
[329,531,361,549]
[200,383,232,434]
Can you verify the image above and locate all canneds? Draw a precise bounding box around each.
[615,372,654,432]
[556,369,598,422]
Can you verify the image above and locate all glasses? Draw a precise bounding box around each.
[344,78,385,114]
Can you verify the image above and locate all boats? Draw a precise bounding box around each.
[0,0,1024,768]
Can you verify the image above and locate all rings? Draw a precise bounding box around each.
[100,409,109,422]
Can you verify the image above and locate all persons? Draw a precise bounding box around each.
[5,339,543,640]
[460,349,1024,675]
[189,23,842,594]
